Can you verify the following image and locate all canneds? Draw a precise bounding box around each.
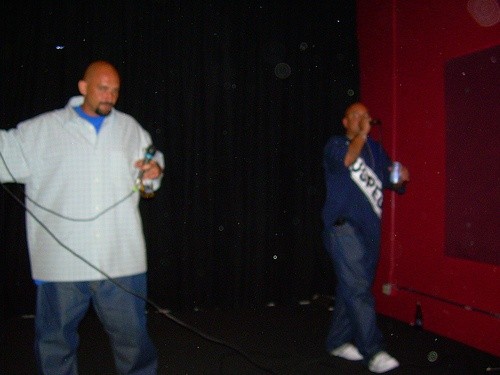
[389,161,402,184]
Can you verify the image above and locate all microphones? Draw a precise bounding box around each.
[136,146,156,184]
[370,119,381,126]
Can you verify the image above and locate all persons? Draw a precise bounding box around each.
[0,61,163,375]
[320,103,408,373]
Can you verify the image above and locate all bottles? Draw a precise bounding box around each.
[415,302,424,328]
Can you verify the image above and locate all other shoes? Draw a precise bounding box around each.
[329,341,364,361]
[368,350,400,373]
